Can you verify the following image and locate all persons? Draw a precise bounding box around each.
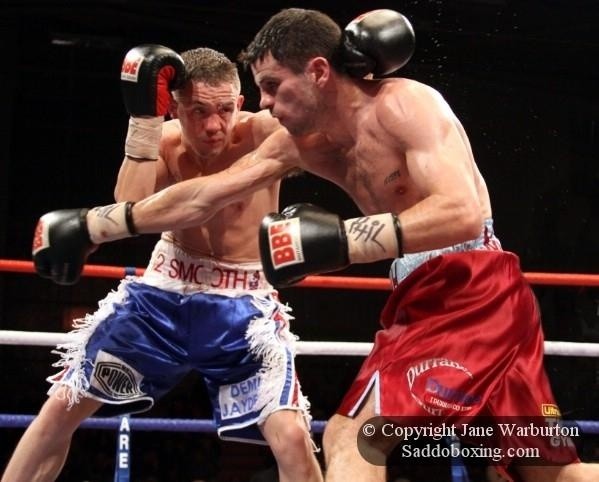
[33,8,599,482]
[0,47,324,482]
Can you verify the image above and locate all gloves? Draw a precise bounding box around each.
[31,200,137,288]
[258,202,404,289]
[337,9,415,81]
[121,45,186,161]
